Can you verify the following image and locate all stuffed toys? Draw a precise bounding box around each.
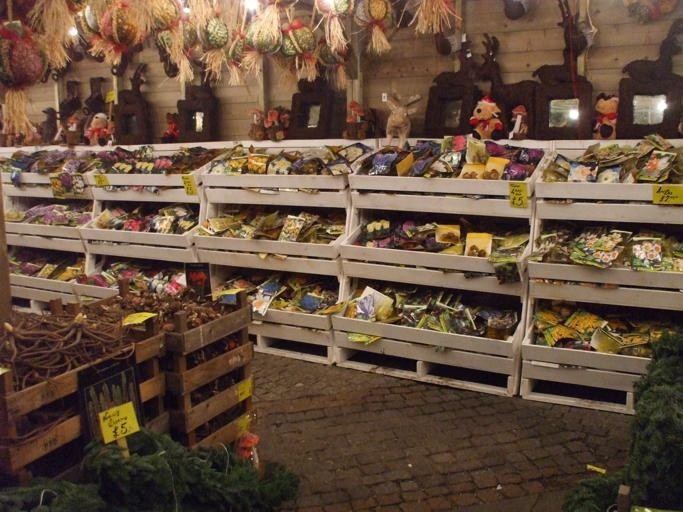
[507,102,529,140]
[1,103,118,146]
[159,111,182,143]
[592,93,620,140]
[469,95,505,141]
[382,87,424,149]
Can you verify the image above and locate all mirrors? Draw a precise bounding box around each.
[632,94,667,126]
[443,100,464,130]
[193,110,205,131]
[306,104,321,129]
[550,99,581,129]
[124,111,138,136]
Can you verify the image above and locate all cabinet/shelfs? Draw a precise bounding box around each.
[0,138,683,416]
